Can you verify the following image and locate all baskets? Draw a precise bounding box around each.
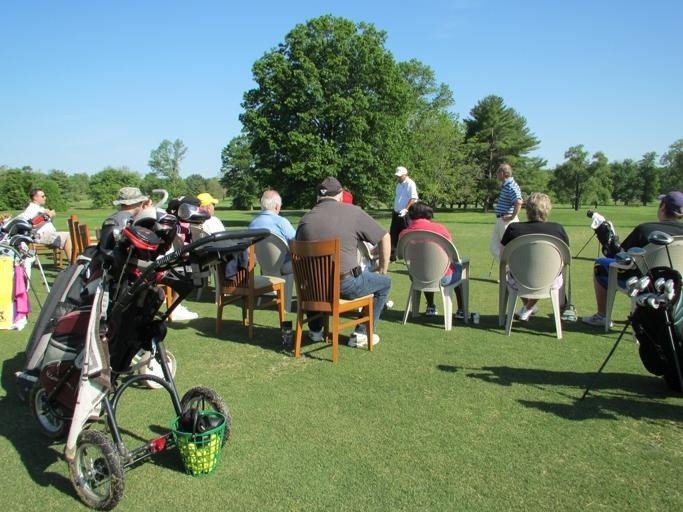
[171,396,227,477]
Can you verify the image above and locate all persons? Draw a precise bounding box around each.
[100,188,231,321]
[390,166,419,262]
[499,192,570,322]
[342,188,394,312]
[490,164,522,284]
[248,190,297,275]
[582,191,682,328]
[398,202,465,320]
[296,176,392,349]
[24,188,71,266]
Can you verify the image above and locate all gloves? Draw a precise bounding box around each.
[398,208,409,217]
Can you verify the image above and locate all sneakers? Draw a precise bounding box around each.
[581,312,613,328]
[347,332,379,347]
[308,327,324,343]
[514,304,537,322]
[454,309,479,321]
[425,303,438,316]
[171,303,198,321]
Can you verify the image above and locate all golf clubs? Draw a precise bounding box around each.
[572,229,596,258]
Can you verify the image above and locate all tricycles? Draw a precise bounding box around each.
[12,215,269,508]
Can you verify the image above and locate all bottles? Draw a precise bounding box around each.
[281,321,293,347]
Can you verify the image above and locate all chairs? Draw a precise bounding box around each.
[153,284,180,325]
[358,241,380,272]
[605,235,683,332]
[74,221,85,255]
[499,233,571,339]
[68,214,81,265]
[210,244,287,338]
[190,226,217,301]
[289,238,374,363]
[396,230,470,331]
[28,242,63,268]
[79,224,101,252]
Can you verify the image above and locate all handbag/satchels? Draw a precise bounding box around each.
[632,264,683,394]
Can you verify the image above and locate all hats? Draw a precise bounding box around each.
[196,193,219,206]
[395,167,407,177]
[657,191,683,207]
[111,186,148,205]
[316,176,342,196]
